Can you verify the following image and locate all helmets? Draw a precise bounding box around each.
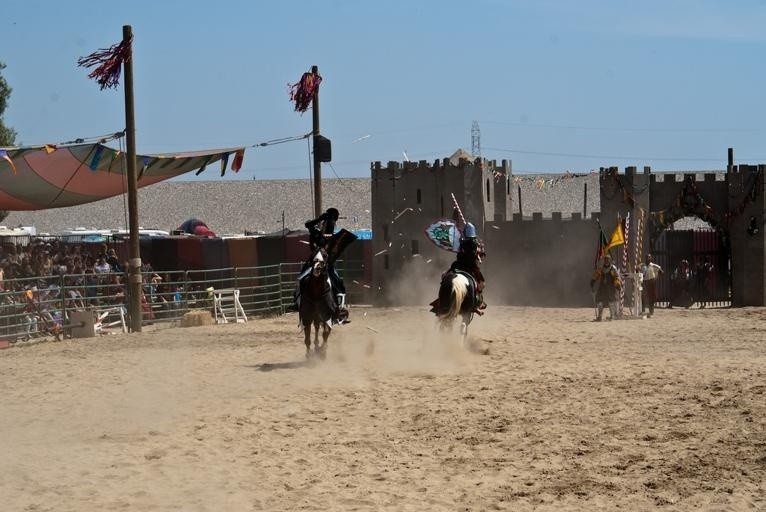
[464,222,477,239]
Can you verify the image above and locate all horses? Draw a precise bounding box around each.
[298,239,338,366]
[437,265,476,342]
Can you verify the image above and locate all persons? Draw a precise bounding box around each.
[0,237,183,341]
[300,207,349,318]
[667,258,693,309]
[441,222,488,310]
[635,253,664,315]
[589,254,623,321]
[692,254,714,309]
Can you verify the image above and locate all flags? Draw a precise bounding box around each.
[604,224,625,253]
[592,227,610,270]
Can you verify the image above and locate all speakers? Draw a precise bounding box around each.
[313,135,331,162]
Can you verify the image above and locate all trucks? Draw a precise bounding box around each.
[0,223,270,248]
